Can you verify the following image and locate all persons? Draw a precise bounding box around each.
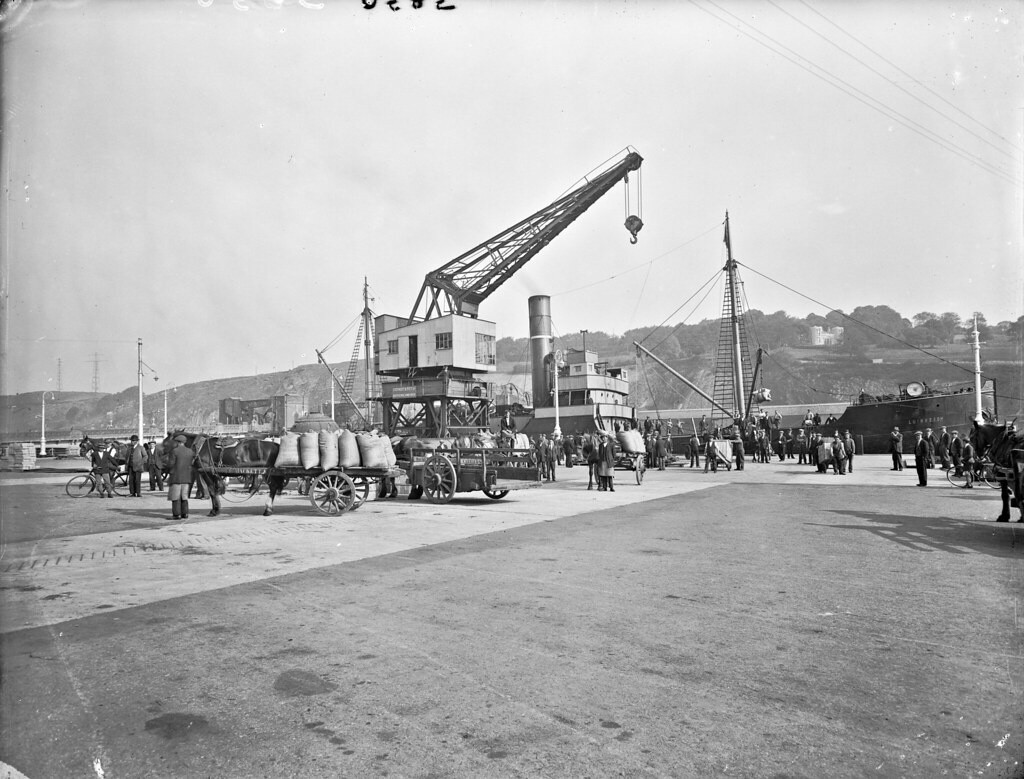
[690,433,701,468]
[797,429,828,472]
[825,414,837,424]
[769,429,795,461]
[501,411,517,449]
[699,410,771,473]
[891,427,903,471]
[530,432,586,482]
[758,409,772,436]
[614,416,684,471]
[167,436,195,519]
[90,435,164,499]
[598,434,616,492]
[587,395,593,404]
[773,409,781,428]
[914,427,973,486]
[831,429,855,475]
[804,409,822,427]
[923,381,933,398]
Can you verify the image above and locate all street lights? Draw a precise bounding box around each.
[39,391,55,455]
[164,382,177,438]
[138,361,159,449]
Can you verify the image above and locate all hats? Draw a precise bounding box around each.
[891,425,972,443]
[130,435,140,441]
[98,443,107,448]
[647,430,850,442]
[600,434,608,437]
[174,435,187,443]
[148,441,156,445]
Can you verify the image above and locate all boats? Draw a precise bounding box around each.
[517,209,998,456]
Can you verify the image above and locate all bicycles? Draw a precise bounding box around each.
[947,461,1002,491]
[65,466,132,498]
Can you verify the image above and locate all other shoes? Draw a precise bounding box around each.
[889,463,976,488]
[659,467,665,471]
[147,488,155,491]
[182,513,188,518]
[129,495,136,497]
[137,495,142,497]
[166,515,182,520]
[599,487,607,491]
[100,493,105,498]
[734,468,744,470]
[610,487,615,491]
[159,487,164,491]
[759,456,853,475]
[108,494,114,498]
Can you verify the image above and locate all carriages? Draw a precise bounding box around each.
[155,429,405,517]
[582,434,648,490]
[377,434,544,504]
